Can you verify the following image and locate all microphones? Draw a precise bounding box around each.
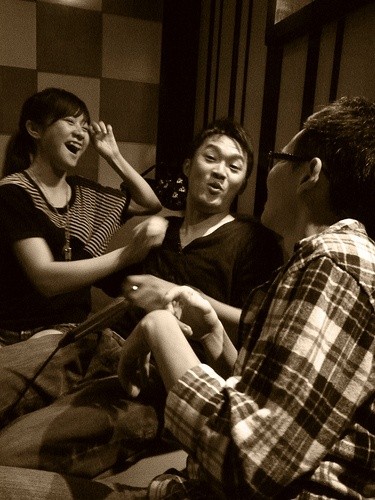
[59,286,138,347]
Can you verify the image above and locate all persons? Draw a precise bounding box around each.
[0,89,163,348]
[0,116,270,469]
[0,93,375,499]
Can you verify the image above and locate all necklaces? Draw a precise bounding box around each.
[29,167,71,260]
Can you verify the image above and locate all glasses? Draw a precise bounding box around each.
[265,150,331,179]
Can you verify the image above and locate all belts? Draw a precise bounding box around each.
[0,326,47,341]
